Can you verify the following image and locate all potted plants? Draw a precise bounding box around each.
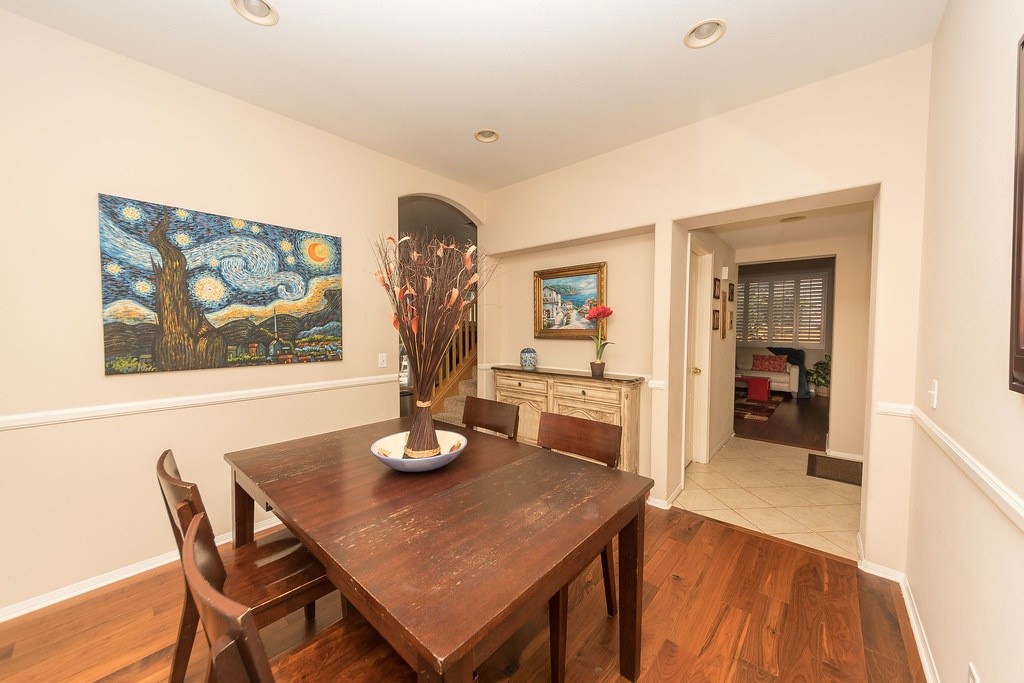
[804,354,830,396]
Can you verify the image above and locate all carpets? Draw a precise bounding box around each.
[807,452,862,485]
[735,392,783,421]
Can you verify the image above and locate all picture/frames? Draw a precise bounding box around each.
[532,260,609,339]
[713,279,721,298]
[728,283,735,302]
[728,311,733,331]
[720,290,728,338]
[713,309,720,330]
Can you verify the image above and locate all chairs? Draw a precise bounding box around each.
[156,447,349,683]
[459,396,519,439]
[506,412,623,683]
[181,510,424,683]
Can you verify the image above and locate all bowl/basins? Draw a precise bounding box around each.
[369,429,468,472]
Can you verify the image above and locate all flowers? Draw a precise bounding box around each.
[587,304,614,362]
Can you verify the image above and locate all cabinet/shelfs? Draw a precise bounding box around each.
[490,365,644,478]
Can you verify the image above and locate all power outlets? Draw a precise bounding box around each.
[967,664,978,683]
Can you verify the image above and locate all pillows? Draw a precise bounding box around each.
[752,354,788,373]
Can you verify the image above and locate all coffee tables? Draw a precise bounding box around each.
[735,375,770,401]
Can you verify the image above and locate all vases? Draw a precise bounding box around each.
[590,362,606,379]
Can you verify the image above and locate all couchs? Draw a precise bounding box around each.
[735,347,802,397]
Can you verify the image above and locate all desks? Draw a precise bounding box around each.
[225,416,656,683]
